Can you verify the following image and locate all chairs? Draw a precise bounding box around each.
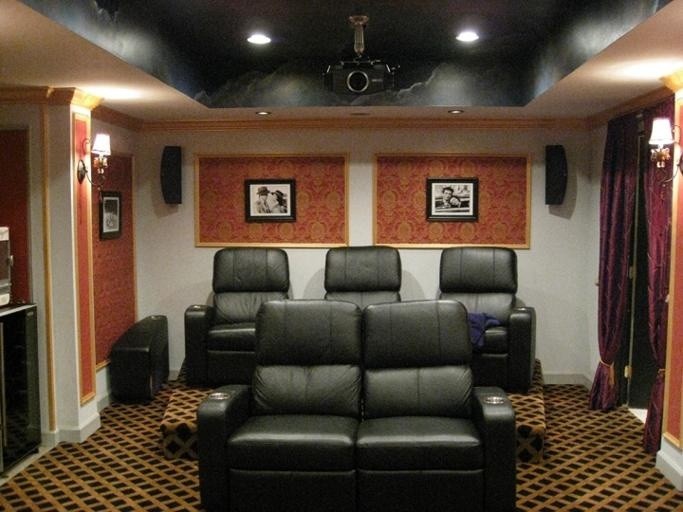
[196,300,360,511]
[355,300,516,512]
[437,247,535,396]
[323,246,401,313]
[184,246,289,389]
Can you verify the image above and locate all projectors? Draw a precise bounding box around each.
[321,60,401,95]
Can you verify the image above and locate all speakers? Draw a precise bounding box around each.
[160,146,181,203]
[546,145,567,204]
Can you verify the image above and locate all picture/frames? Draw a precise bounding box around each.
[245,179,297,222]
[426,178,478,222]
[101,192,121,239]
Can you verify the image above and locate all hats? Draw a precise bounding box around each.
[271,190,284,199]
[256,186,270,194]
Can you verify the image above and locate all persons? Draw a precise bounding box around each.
[253,187,271,213]
[435,188,454,211]
[268,190,286,214]
[447,196,469,212]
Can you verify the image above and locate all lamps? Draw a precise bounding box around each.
[78,134,111,192]
[647,117,682,184]
